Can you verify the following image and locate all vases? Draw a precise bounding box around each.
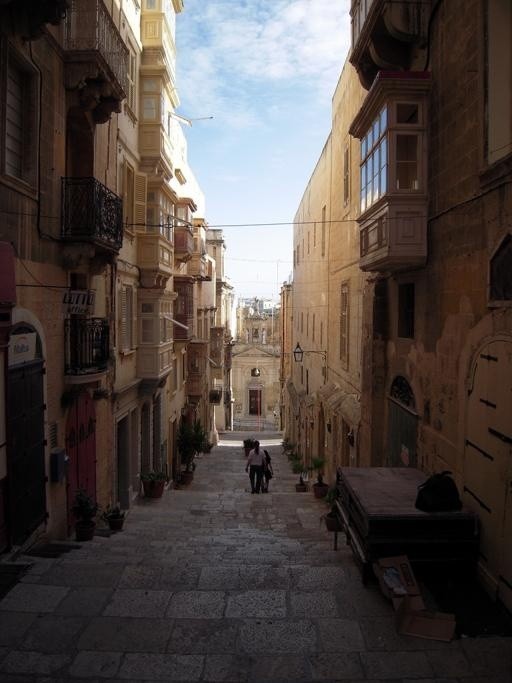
[326,510,344,531]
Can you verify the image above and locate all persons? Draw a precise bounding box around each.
[244,440,266,494]
[261,450,273,489]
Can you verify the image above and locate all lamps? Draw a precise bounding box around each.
[293,342,327,362]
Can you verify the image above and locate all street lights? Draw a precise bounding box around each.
[254,359,280,376]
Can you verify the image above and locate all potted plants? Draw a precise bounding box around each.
[243,438,255,455]
[140,471,168,498]
[100,507,126,530]
[312,457,330,498]
[71,493,99,541]
[292,463,308,492]
[176,419,206,485]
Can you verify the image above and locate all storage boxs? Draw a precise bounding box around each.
[374,551,457,642]
